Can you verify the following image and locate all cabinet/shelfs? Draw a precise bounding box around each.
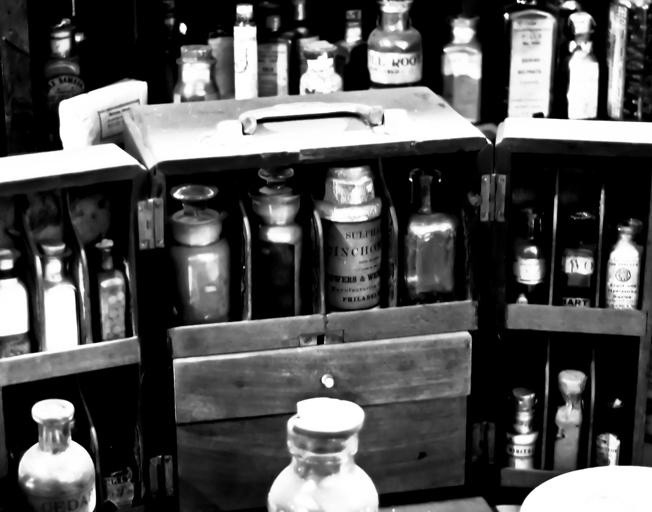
[0,85,652,512]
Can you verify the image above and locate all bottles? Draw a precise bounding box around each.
[504,387,541,470]
[1,195,127,359]
[168,184,233,323]
[608,216,644,309]
[408,169,457,302]
[509,202,548,307]
[18,398,99,512]
[314,162,384,313]
[552,367,589,472]
[561,212,596,311]
[268,397,380,510]
[42,1,652,127]
[250,165,305,319]
[588,388,626,469]
